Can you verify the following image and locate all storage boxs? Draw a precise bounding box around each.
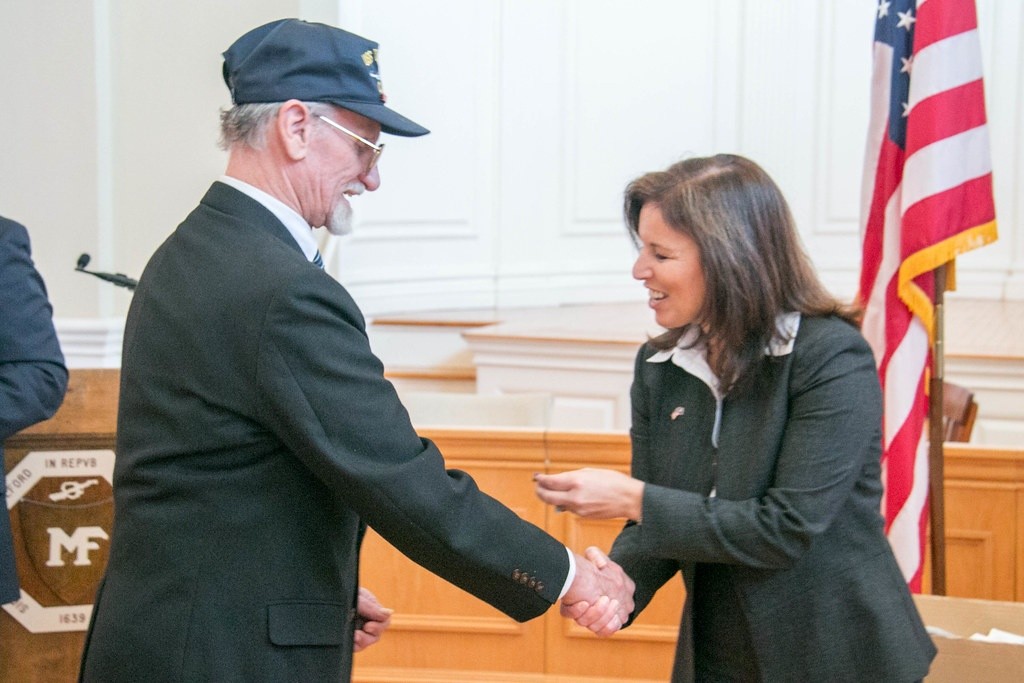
[913,594,1024,683]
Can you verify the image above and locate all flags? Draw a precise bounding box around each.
[851,0,1002,592]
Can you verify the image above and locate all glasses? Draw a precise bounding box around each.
[314,114,385,173]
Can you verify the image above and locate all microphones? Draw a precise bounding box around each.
[78,254,90,268]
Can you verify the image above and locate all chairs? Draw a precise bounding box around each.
[942,382,978,443]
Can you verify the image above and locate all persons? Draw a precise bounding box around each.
[534,154,937,683]
[78,18,637,683]
[0,216,69,603]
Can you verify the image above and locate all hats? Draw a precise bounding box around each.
[219,17,431,138]
